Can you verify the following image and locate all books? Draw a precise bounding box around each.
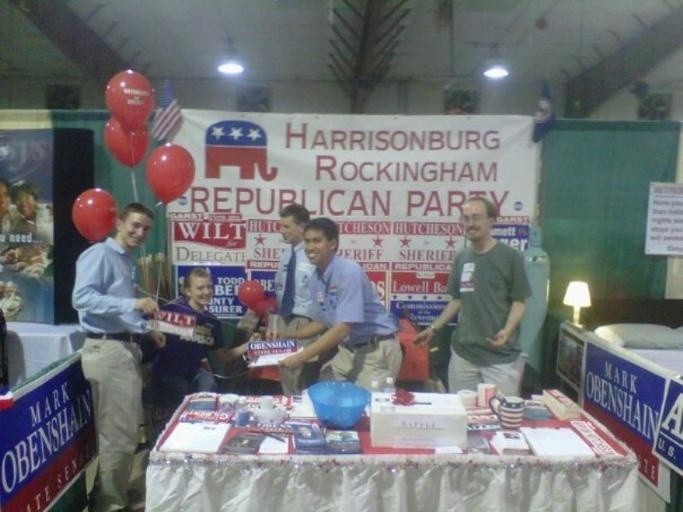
[223,430,265,454]
[323,428,361,454]
[292,424,327,456]
[290,394,319,421]
[489,430,530,457]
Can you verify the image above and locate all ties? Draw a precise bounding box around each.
[279,249,296,321]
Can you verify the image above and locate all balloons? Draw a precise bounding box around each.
[237,282,265,308]
[104,68,155,131]
[71,188,118,244]
[146,142,196,205]
[101,119,150,166]
[267,294,279,307]
[254,300,276,321]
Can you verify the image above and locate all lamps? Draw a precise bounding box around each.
[215,34,245,76]
[477,41,514,81]
[558,279,594,332]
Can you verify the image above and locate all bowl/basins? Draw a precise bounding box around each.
[307,381,369,430]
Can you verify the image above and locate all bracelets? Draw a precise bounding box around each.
[427,324,440,336]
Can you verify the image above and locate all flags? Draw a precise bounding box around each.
[148,74,187,144]
[532,80,557,144]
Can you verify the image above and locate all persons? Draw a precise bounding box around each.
[272,218,403,391]
[72,202,166,512]
[412,196,533,399]
[0,175,55,279]
[148,268,263,395]
[273,204,325,396]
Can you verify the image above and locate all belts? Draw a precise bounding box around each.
[353,333,394,348]
[85,332,141,343]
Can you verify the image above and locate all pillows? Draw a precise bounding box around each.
[591,322,683,351]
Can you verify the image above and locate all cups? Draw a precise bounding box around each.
[489,396,525,430]
[478,383,496,408]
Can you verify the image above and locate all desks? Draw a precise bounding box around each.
[141,387,641,512]
